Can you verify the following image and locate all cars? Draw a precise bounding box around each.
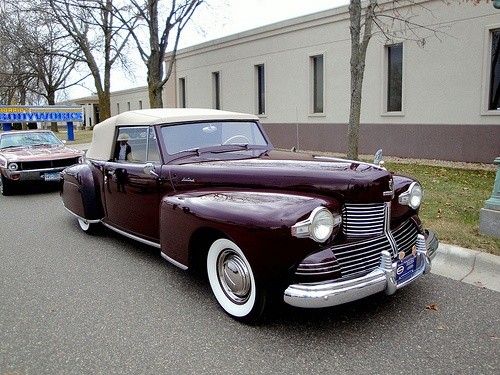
[0,130,86,195]
[58,107,438,321]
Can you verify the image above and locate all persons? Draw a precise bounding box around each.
[113,133,134,162]
[112,168,130,194]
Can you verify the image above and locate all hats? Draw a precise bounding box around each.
[117,134,129,140]
